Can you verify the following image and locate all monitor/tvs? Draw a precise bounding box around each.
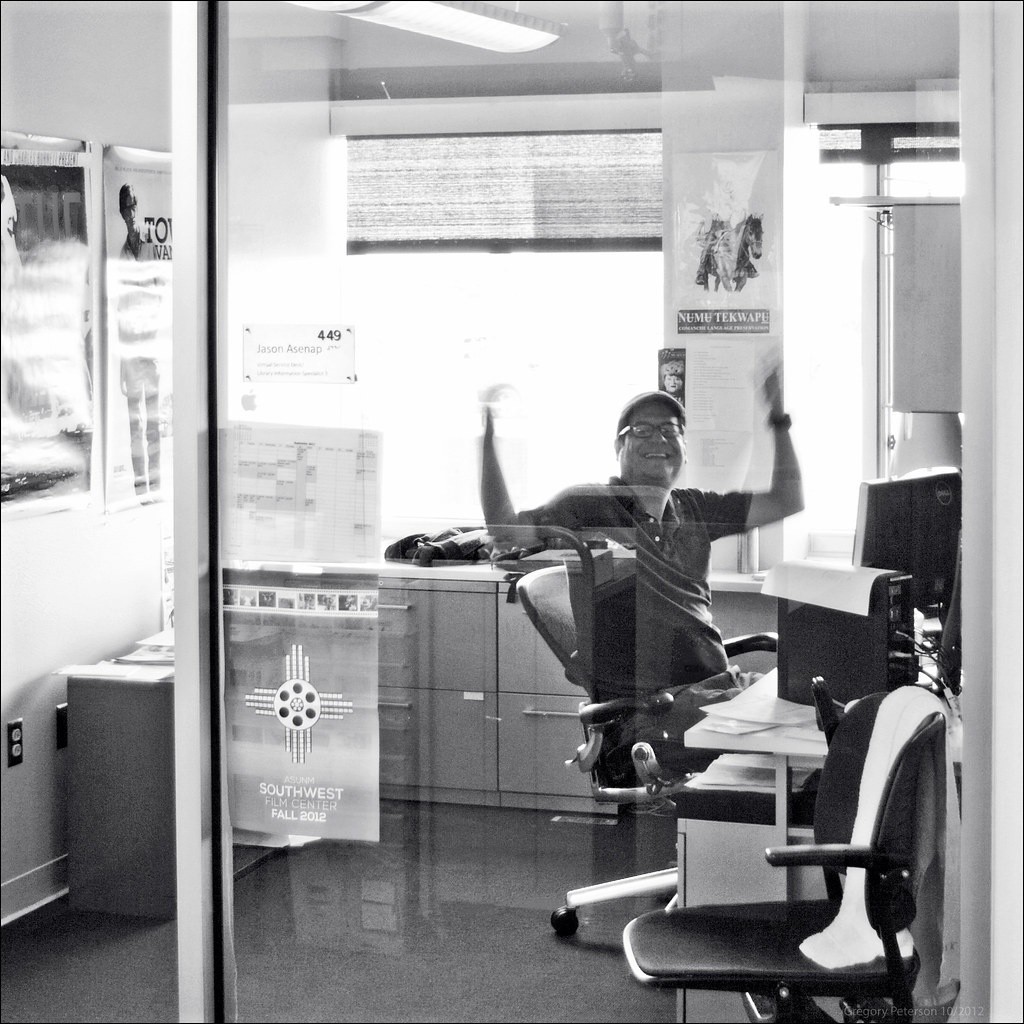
[851,465,962,609]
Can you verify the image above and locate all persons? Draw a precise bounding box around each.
[470,344,808,782]
[119,183,158,499]
[658,356,686,403]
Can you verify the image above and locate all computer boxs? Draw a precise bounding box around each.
[777,570,916,707]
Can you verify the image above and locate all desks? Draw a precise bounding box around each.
[677,666,963,1021]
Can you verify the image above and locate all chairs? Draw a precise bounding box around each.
[623,691,946,1023]
[516,565,779,933]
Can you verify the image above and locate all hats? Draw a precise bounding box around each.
[618,389,688,428]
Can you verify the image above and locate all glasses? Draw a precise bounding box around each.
[618,425,685,439]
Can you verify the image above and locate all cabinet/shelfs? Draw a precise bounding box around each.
[294,560,779,816]
[68,648,177,919]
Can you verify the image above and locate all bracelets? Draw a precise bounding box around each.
[766,411,792,433]
